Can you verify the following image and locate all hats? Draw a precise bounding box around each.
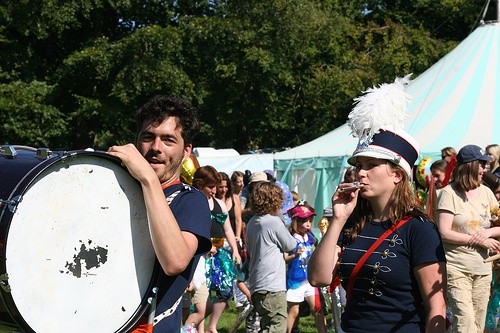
[458,144,493,162]
[248,171,268,182]
[264,170,274,177]
[291,206,317,218]
[347,73,418,178]
[323,208,334,217]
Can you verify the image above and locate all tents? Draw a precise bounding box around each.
[193,21,500,239]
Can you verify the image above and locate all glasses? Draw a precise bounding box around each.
[442,154,449,159]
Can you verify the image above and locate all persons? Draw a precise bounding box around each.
[179,144,500,333]
[105,94,211,333]
[304,74,450,333]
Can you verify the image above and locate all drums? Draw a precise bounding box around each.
[0,145,160,333]
